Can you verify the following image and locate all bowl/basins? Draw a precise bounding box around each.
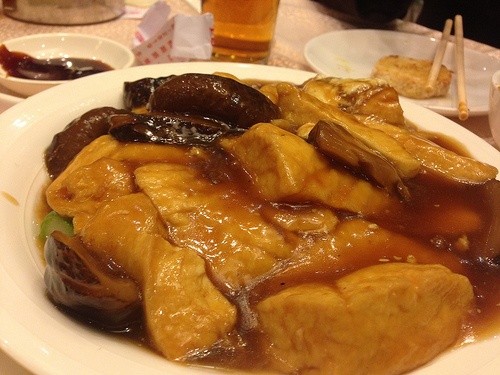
[1,33,134,97]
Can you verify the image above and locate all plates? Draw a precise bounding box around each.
[0,62,500,372]
[304,28,500,118]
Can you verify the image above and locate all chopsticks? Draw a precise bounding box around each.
[427,14,470,122]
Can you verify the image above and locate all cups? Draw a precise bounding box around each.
[201,1,280,64]
